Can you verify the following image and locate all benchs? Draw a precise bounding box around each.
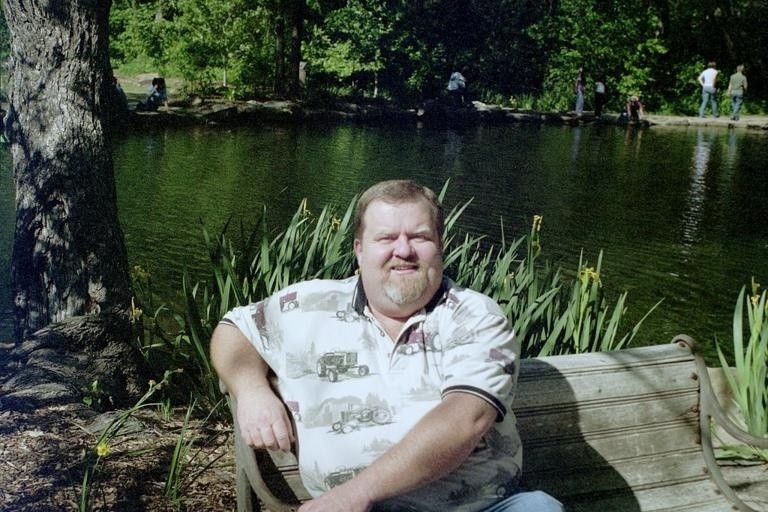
[218,332,767,512]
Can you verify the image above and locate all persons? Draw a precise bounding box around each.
[209,178,566,512]
[446,66,469,101]
[627,96,643,120]
[726,64,748,120]
[697,61,720,117]
[594,82,606,120]
[147,71,167,102]
[574,67,585,114]
